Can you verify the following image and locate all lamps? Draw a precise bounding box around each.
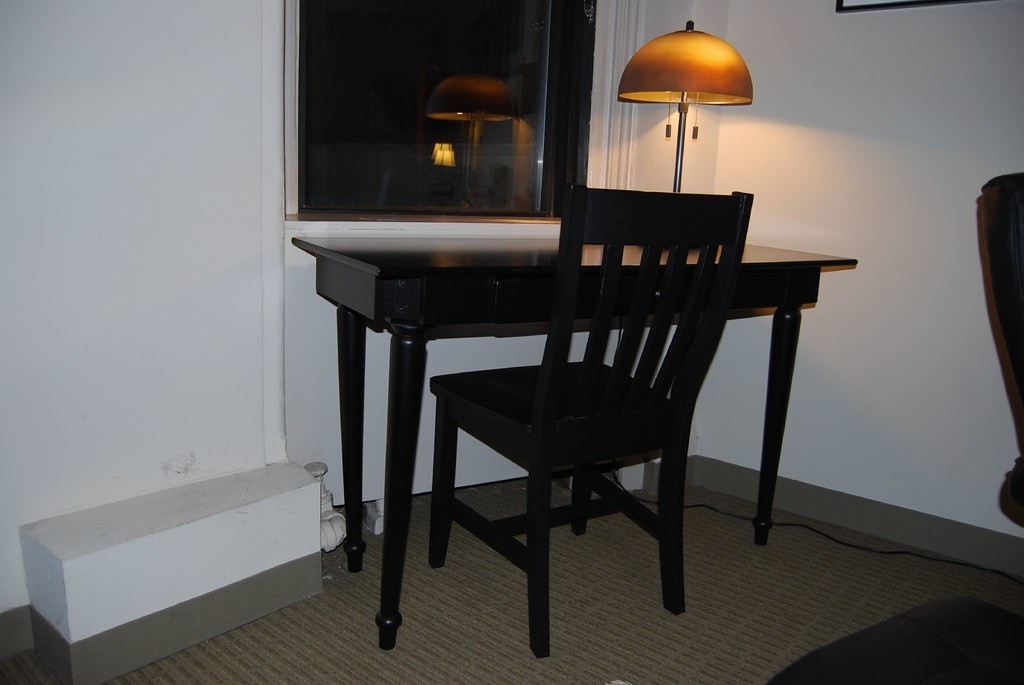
[425,73,517,171]
[616,17,754,193]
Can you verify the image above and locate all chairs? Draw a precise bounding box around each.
[975,170,1024,528]
[427,186,755,659]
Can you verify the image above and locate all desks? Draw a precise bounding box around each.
[292,235,858,651]
